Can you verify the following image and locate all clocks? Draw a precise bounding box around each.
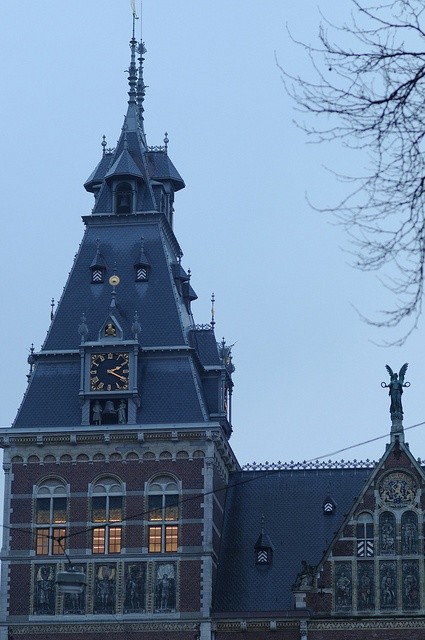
[90,352,128,391]
